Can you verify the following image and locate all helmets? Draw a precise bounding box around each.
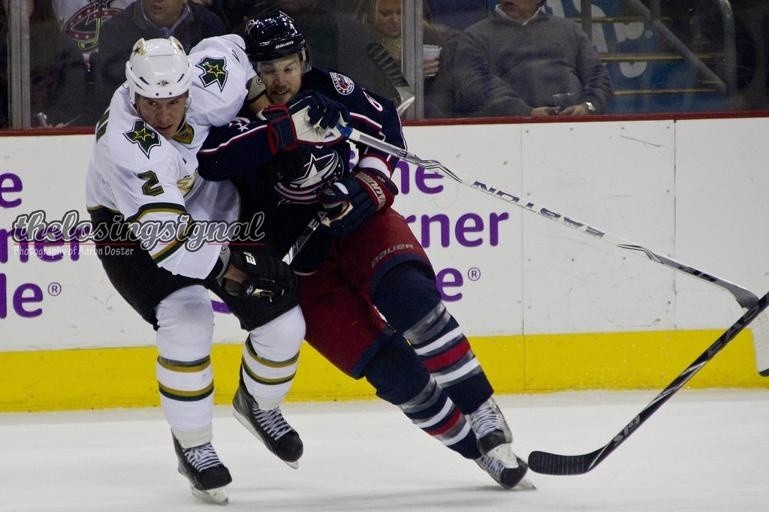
[244,14,306,61]
[125,36,193,104]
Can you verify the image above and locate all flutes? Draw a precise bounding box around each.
[246,95,415,301]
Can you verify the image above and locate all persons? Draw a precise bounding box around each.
[197,9,530,491]
[87,34,308,492]
[1,2,767,132]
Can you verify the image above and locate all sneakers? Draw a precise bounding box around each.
[172,433,232,490]
[463,397,527,489]
[233,386,303,461]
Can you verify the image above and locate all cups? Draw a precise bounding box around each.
[421,44,441,78]
[552,91,575,115]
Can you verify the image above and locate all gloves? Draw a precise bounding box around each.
[224,252,296,310]
[262,92,351,155]
[316,169,398,232]
